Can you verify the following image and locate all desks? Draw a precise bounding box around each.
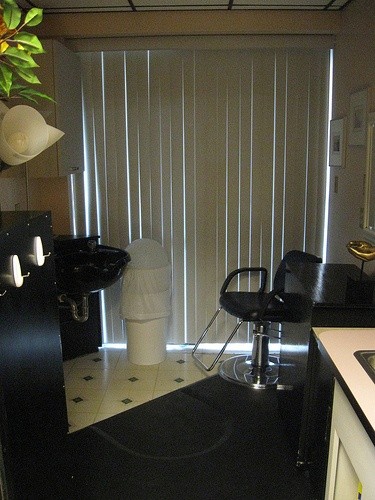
[277,263,375,471]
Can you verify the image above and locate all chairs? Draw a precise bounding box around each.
[191,249,322,390]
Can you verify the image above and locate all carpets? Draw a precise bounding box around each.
[69,374,321,500]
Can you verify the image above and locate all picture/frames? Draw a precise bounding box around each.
[328,115,348,169]
[362,108,375,234]
[347,86,371,148]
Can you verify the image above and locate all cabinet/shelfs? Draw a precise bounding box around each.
[310,327,375,500]
[25,39,84,181]
[0,210,69,500]
[54,235,102,361]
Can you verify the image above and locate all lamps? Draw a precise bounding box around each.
[0,100,66,172]
